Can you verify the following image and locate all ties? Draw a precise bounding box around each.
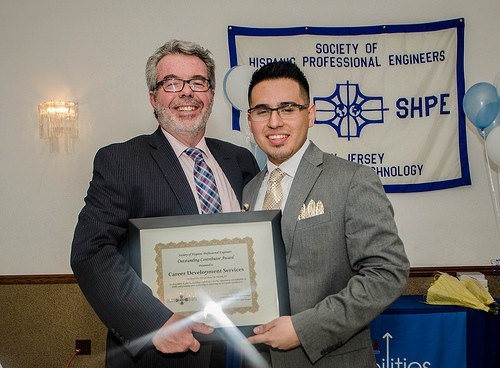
[262,168,286,210]
[184,148,223,214]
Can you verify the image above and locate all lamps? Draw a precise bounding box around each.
[38,102,79,138]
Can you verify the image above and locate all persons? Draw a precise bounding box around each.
[70,40,261,368]
[240,61,410,368]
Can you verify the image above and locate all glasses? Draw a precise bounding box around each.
[153,79,213,92]
[248,105,308,121]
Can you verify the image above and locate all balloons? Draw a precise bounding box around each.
[222,65,256,144]
[462,82,500,129]
[475,102,500,167]
